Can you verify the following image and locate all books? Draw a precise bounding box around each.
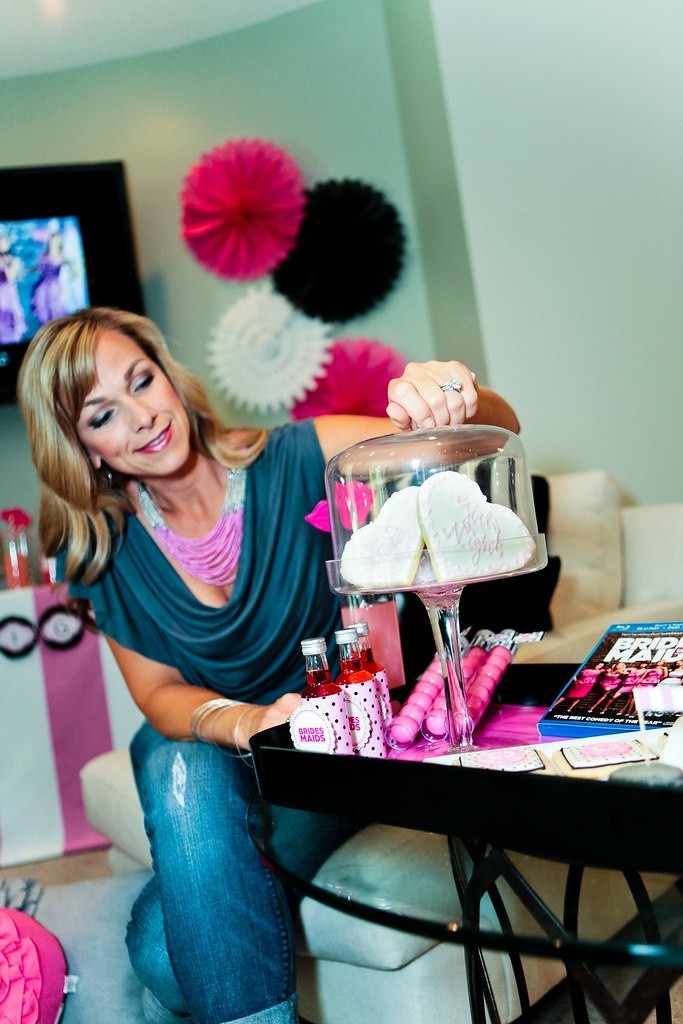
[537,622,683,738]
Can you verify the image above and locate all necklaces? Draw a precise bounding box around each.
[132,432,247,586]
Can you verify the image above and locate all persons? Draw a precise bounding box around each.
[548,661,611,712]
[626,659,666,718]
[600,660,651,715]
[16,308,520,1024]
[588,662,626,714]
[653,661,683,713]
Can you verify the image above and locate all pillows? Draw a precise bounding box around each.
[33,868,161,1024]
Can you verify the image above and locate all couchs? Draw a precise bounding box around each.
[81,472,683,1024]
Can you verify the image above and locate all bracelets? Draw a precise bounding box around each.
[187,696,253,768]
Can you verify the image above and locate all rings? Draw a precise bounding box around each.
[439,379,462,392]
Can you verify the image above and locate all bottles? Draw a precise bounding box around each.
[334,629,387,758]
[344,623,392,728]
[299,638,356,754]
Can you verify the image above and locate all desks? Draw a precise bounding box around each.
[248,663,683,1024]
